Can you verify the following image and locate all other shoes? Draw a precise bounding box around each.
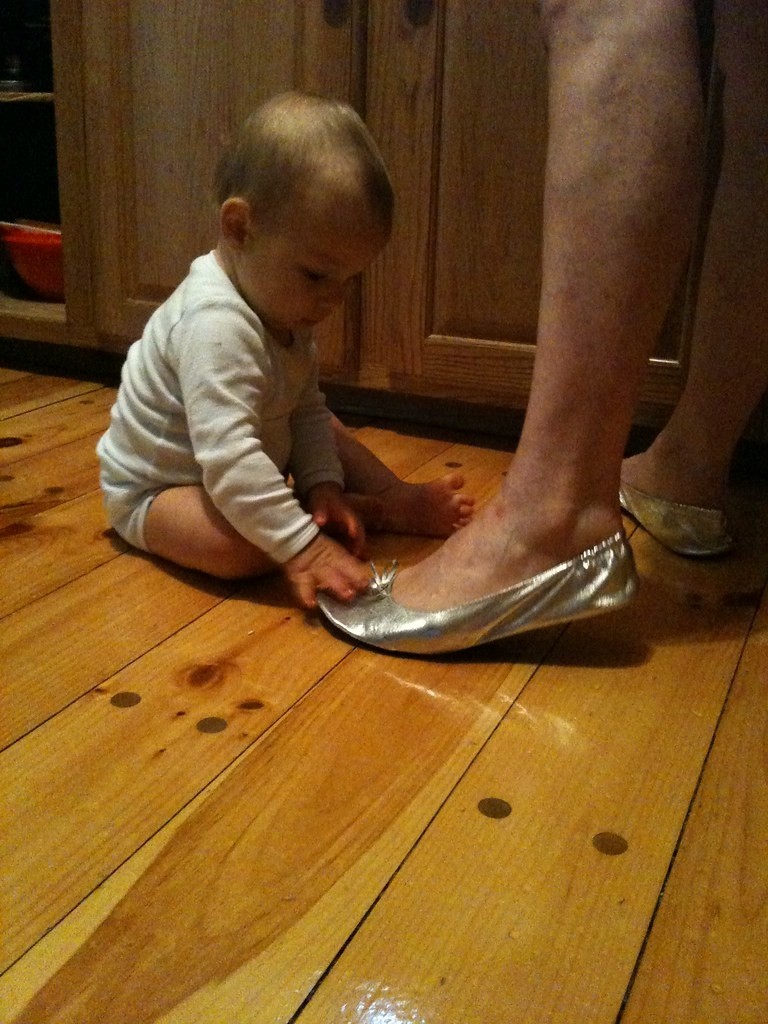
[620,481,738,558]
[315,526,639,662]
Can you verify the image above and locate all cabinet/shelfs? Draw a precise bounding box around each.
[0,0,93,348]
[80,0,768,445]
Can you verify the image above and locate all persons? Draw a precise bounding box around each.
[95,92,475,610]
[316,0,768,655]
